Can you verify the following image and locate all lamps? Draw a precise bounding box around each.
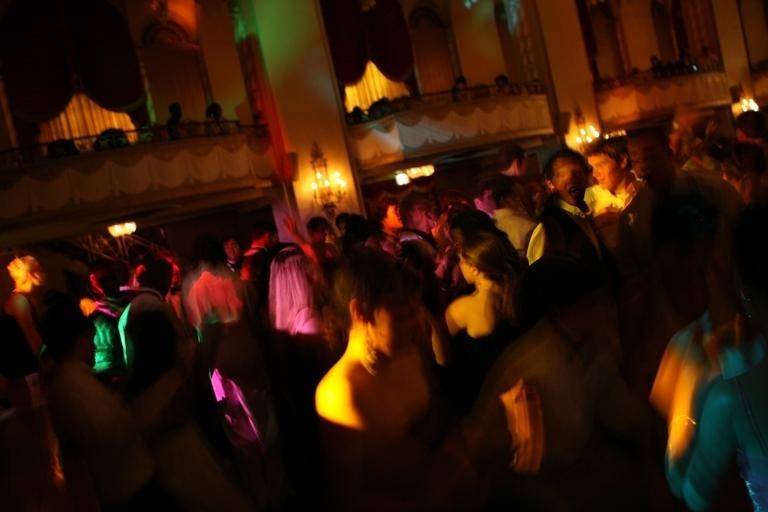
[309,143,347,217]
[730,82,759,112]
[574,105,600,154]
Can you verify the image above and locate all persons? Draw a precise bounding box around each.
[1,40,768,511]
